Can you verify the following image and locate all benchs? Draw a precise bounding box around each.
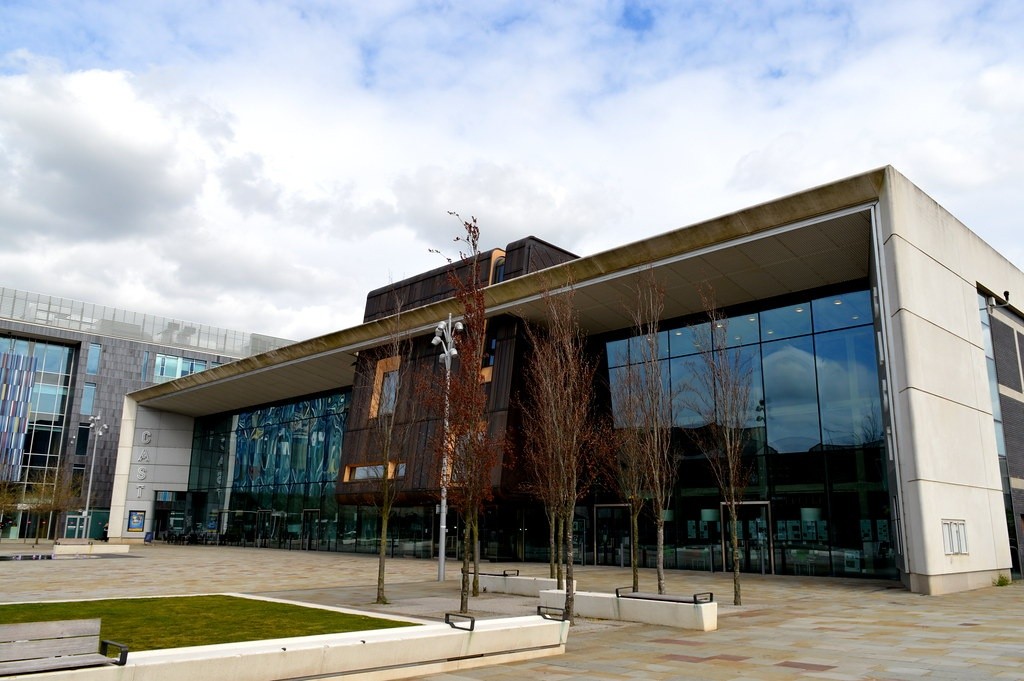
[53,542,93,554]
[460,568,520,593]
[617,586,718,632]
[443,606,571,669]
[0,617,136,681]
